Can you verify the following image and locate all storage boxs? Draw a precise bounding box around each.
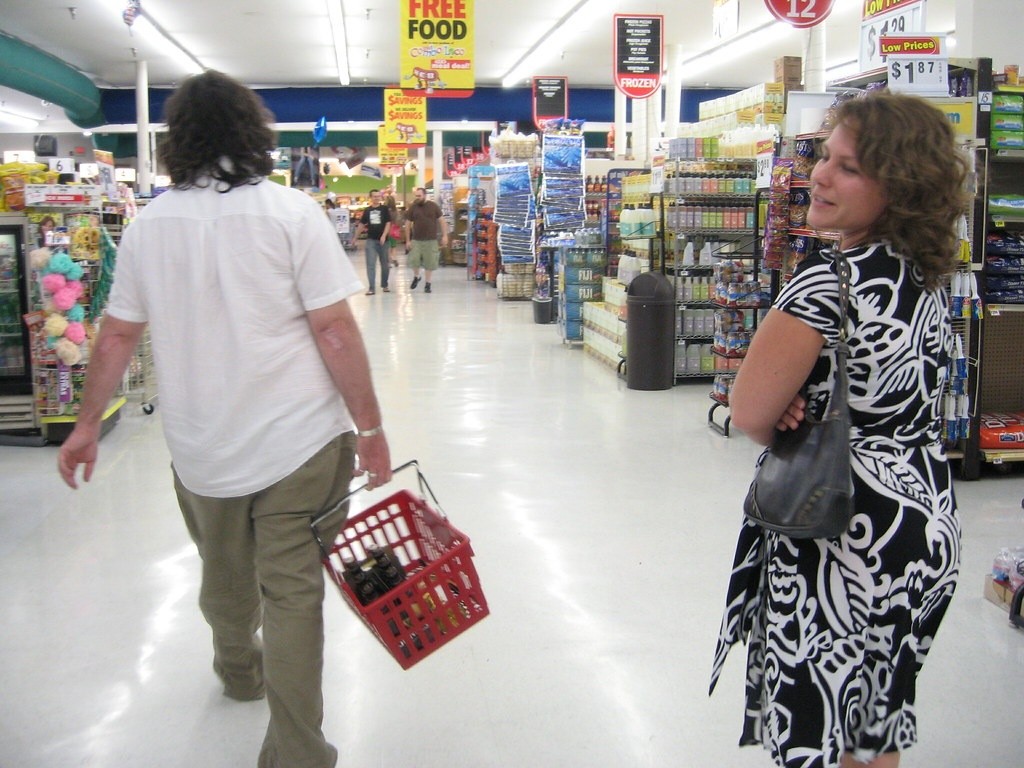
[785,83,805,113]
[990,130,1024,150]
[990,113,1024,132]
[982,573,1024,617]
[774,56,803,84]
[980,410,1024,449]
[991,94,1024,115]
[556,244,630,376]
[988,193,1024,216]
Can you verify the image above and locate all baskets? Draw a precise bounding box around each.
[310,459,490,670]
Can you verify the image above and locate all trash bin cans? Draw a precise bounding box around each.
[625,270,677,391]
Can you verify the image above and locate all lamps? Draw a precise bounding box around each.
[325,0,352,87]
[822,31,957,80]
[661,19,794,86]
[124,0,206,74]
[501,0,602,89]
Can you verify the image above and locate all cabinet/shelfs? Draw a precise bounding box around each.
[477,207,505,288]
[707,232,777,438]
[0,200,128,448]
[583,168,652,278]
[650,157,755,388]
[944,54,1024,483]
[784,129,844,282]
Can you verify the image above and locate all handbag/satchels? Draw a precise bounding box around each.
[742,250,854,539]
[389,224,401,240]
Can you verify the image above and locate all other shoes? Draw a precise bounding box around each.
[383,287,390,292]
[410,276,421,289]
[365,292,375,295]
[424,282,432,293]
[391,259,399,267]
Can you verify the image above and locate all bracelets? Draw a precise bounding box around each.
[358,424,383,437]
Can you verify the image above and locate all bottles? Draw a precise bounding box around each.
[535,227,604,297]
[699,237,754,265]
[344,543,467,659]
[0,242,25,376]
[993,545,1024,584]
[712,261,762,403]
[683,240,694,266]
[666,200,754,230]
[585,174,622,222]
[618,202,657,238]
[664,122,780,195]
[674,269,715,374]
[617,248,641,286]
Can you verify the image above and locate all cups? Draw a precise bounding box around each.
[1004,64,1020,85]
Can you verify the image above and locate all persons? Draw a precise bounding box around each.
[729,94,976,768]
[57,71,391,768]
[404,188,448,293]
[350,189,391,296]
[325,199,337,227]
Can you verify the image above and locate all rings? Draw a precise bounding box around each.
[358,469,365,473]
[369,472,377,478]
